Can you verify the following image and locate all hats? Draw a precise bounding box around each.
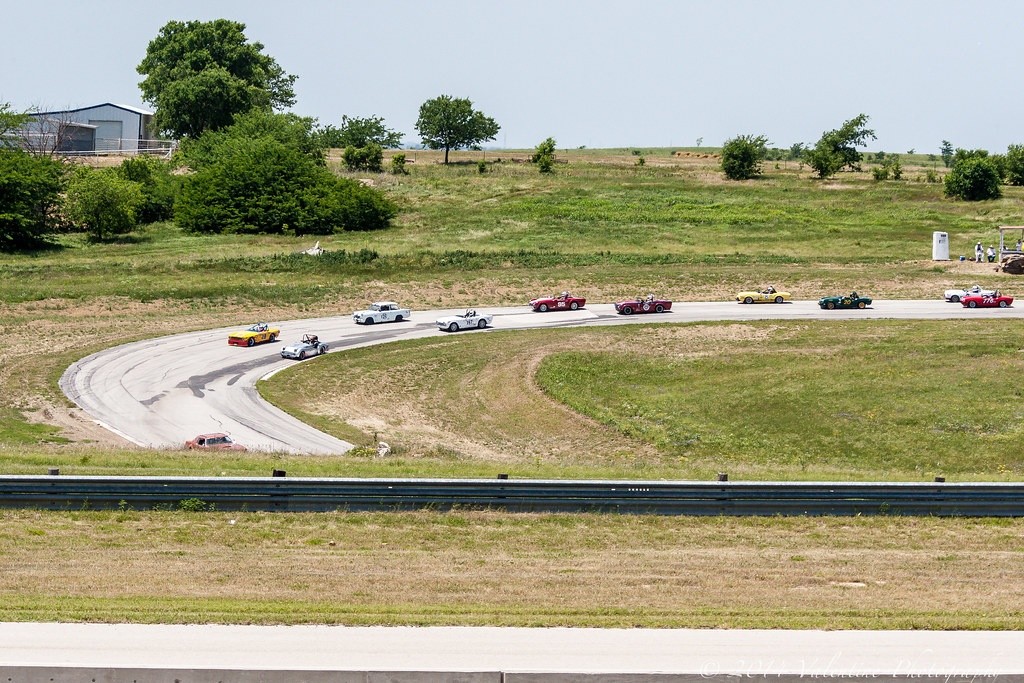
[989,245,994,247]
[978,242,980,244]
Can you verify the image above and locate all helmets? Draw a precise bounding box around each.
[646,295,652,300]
[310,336,314,342]
[850,293,853,297]
[972,286,979,291]
[561,292,566,296]
[470,311,474,315]
[768,288,772,292]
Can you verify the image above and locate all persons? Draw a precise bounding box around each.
[468,311,474,318]
[309,337,318,348]
[559,292,566,300]
[974,242,984,263]
[259,326,265,333]
[987,245,996,262]
[1016,239,1021,251]
[646,296,652,303]
[768,287,773,294]
[850,293,854,299]
